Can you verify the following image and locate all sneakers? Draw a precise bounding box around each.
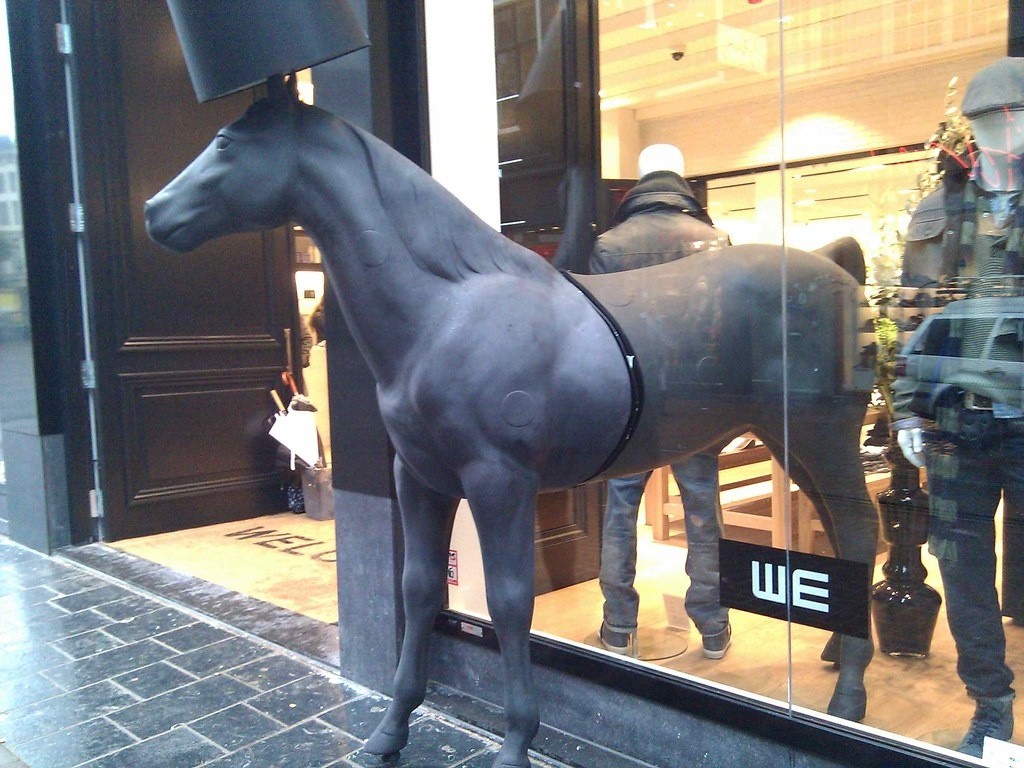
[598,621,630,655]
[703,623,732,659]
[954,699,1014,758]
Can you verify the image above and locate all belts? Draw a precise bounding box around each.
[935,385,993,410]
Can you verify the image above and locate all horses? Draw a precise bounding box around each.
[143,67,877,768]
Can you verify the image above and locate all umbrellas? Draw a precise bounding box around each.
[269,371,327,472]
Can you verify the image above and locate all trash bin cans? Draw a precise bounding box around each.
[301,466,337,522]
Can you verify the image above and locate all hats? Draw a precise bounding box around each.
[960,57,1024,120]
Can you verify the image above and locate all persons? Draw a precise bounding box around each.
[893,54,1024,762]
[587,143,735,661]
